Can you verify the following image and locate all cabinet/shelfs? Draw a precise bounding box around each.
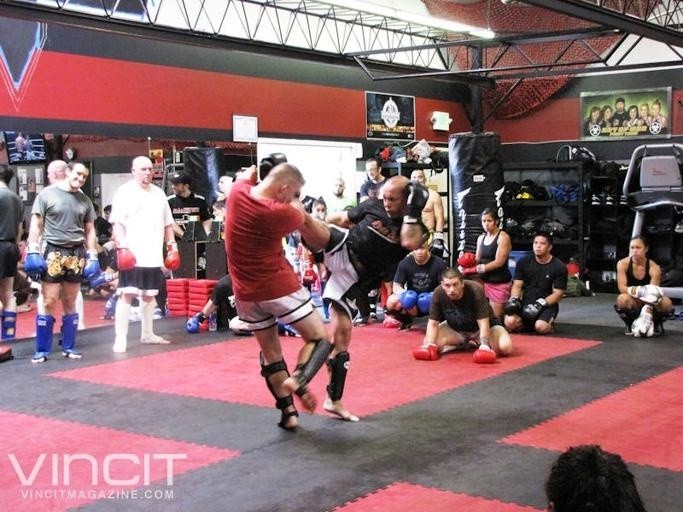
[499,158,587,291]
[587,170,683,295]
[372,159,452,268]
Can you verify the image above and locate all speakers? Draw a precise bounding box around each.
[170,241,208,279]
[205,242,229,280]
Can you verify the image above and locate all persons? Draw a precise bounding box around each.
[13,132,35,162]
[586,97,671,135]
[87,202,119,298]
[28,160,86,333]
[611,237,675,338]
[0,163,26,341]
[296,164,430,423]
[412,268,507,364]
[458,208,512,319]
[106,156,180,355]
[27,160,100,363]
[211,171,236,217]
[404,168,447,261]
[227,162,331,432]
[546,445,643,508]
[299,198,332,326]
[186,273,253,338]
[499,233,569,332]
[353,156,395,328]
[162,170,211,253]
[386,240,448,333]
[323,177,356,219]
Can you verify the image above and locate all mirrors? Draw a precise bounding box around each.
[0,127,257,342]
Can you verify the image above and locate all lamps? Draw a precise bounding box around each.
[312,0,499,44]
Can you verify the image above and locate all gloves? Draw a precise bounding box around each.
[628,285,664,306]
[399,290,417,308]
[523,298,547,319]
[403,182,429,224]
[186,313,209,334]
[24,244,47,273]
[625,304,655,337]
[117,242,136,271]
[461,264,485,274]
[260,153,287,180]
[89,272,113,287]
[458,253,475,267]
[413,343,438,360]
[428,232,444,258]
[418,291,433,313]
[473,344,497,363]
[165,241,181,270]
[504,296,521,316]
[83,251,99,280]
[303,269,318,285]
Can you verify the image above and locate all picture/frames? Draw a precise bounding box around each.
[577,85,674,141]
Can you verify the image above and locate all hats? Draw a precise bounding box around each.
[166,172,192,184]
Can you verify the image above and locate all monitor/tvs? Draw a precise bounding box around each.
[3,130,48,165]
